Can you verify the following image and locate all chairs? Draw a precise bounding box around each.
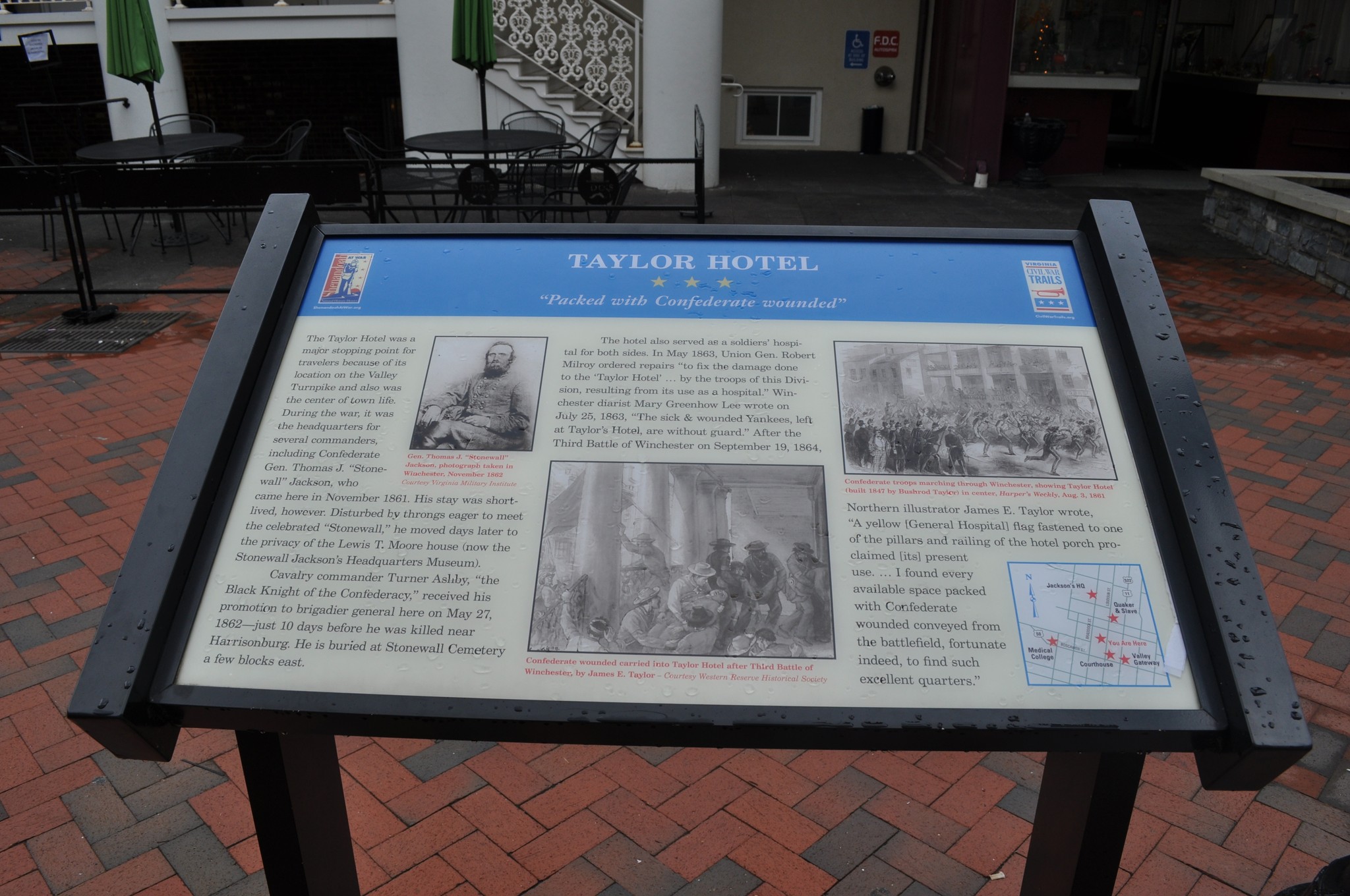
[0,97,319,324]
[339,111,639,230]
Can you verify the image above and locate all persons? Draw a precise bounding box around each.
[410,340,531,452]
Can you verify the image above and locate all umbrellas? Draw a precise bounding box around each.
[105,0,185,233]
[450,0,498,221]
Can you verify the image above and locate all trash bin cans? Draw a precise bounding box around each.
[862,107,883,154]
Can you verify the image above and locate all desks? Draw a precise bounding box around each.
[75,132,245,246]
[404,128,566,223]
